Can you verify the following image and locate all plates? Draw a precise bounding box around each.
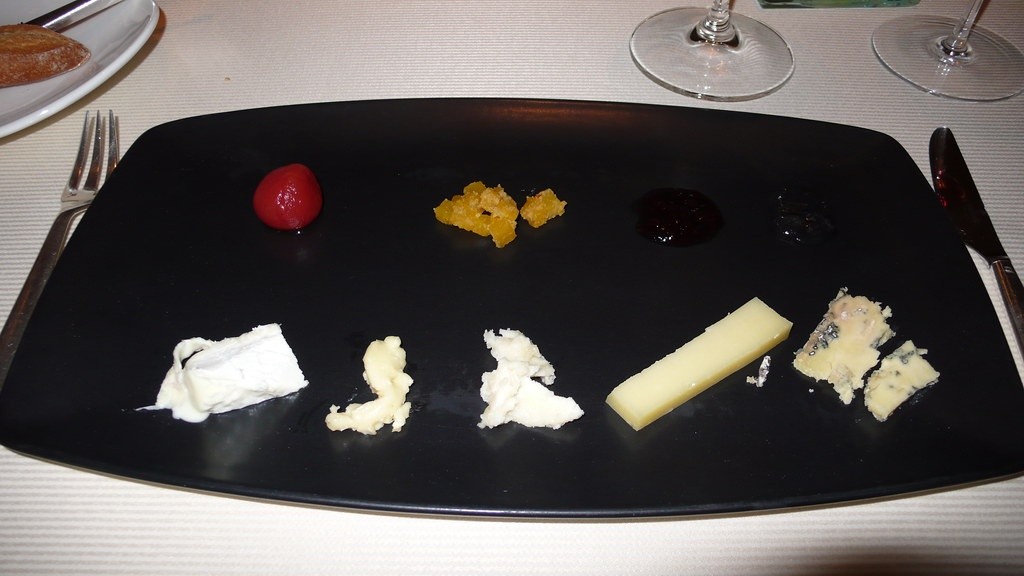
[0,0,159,145]
[0,93,1024,515]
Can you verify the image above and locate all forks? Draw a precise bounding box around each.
[0,106,116,385]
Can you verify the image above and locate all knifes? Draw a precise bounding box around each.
[928,124,1023,349]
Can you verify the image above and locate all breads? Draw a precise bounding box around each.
[0,23,91,90]
